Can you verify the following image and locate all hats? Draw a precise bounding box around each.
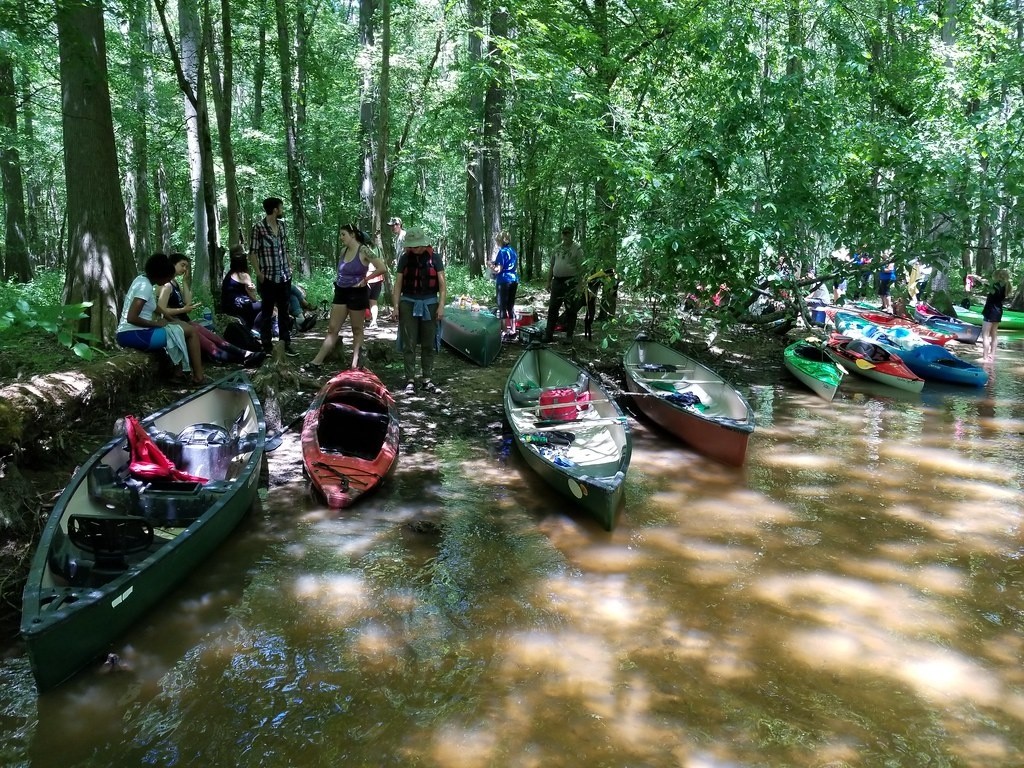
[387,216,402,226]
[403,226,431,248]
[495,230,511,245]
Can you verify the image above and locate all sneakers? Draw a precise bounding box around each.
[192,373,216,388]
[263,345,273,357]
[283,346,300,357]
[237,351,266,368]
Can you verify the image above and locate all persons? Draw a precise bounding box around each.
[385,217,407,322]
[116,253,213,386]
[486,232,519,339]
[155,254,267,368]
[541,227,583,345]
[302,223,386,370]
[968,270,1013,362]
[249,198,300,357]
[682,246,895,337]
[360,232,384,330]
[391,227,446,395]
[218,245,318,339]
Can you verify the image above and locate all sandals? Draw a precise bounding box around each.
[420,379,442,394]
[404,381,416,394]
[298,361,322,375]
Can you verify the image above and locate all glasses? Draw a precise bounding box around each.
[345,223,354,230]
[409,246,420,249]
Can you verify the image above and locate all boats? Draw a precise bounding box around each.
[20,367,268,694]
[622,328,756,469]
[438,299,503,367]
[783,338,846,403]
[805,303,1024,393]
[504,337,633,532]
[300,364,404,510]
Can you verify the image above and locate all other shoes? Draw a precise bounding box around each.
[878,306,894,315]
[365,324,379,330]
[300,314,317,332]
[566,333,574,344]
[541,336,555,343]
[303,303,317,312]
